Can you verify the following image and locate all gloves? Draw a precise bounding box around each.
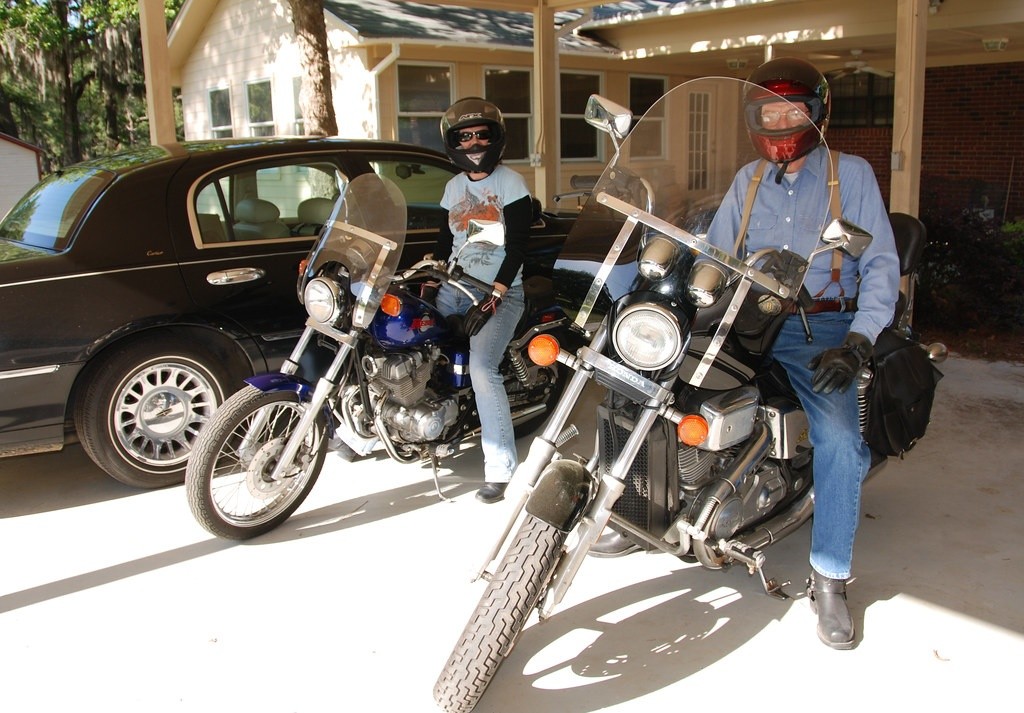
[463,297,502,337]
[807,332,874,395]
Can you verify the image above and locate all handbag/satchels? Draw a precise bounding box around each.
[865,325,945,459]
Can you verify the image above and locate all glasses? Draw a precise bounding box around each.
[759,107,811,127]
[455,130,493,142]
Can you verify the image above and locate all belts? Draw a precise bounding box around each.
[782,296,858,315]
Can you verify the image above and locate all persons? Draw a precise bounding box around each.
[434,96,533,504]
[696,58,900,650]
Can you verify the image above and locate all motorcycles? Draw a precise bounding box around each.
[184,172,604,542]
[431,74,948,713]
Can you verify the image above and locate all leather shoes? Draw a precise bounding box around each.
[809,569,856,650]
[586,531,643,558]
[476,481,509,503]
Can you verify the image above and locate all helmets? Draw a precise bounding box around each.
[742,56,832,162]
[440,96,506,171]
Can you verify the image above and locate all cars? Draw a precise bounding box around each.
[1,138,653,492]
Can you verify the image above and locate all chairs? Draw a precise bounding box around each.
[233,197,338,240]
